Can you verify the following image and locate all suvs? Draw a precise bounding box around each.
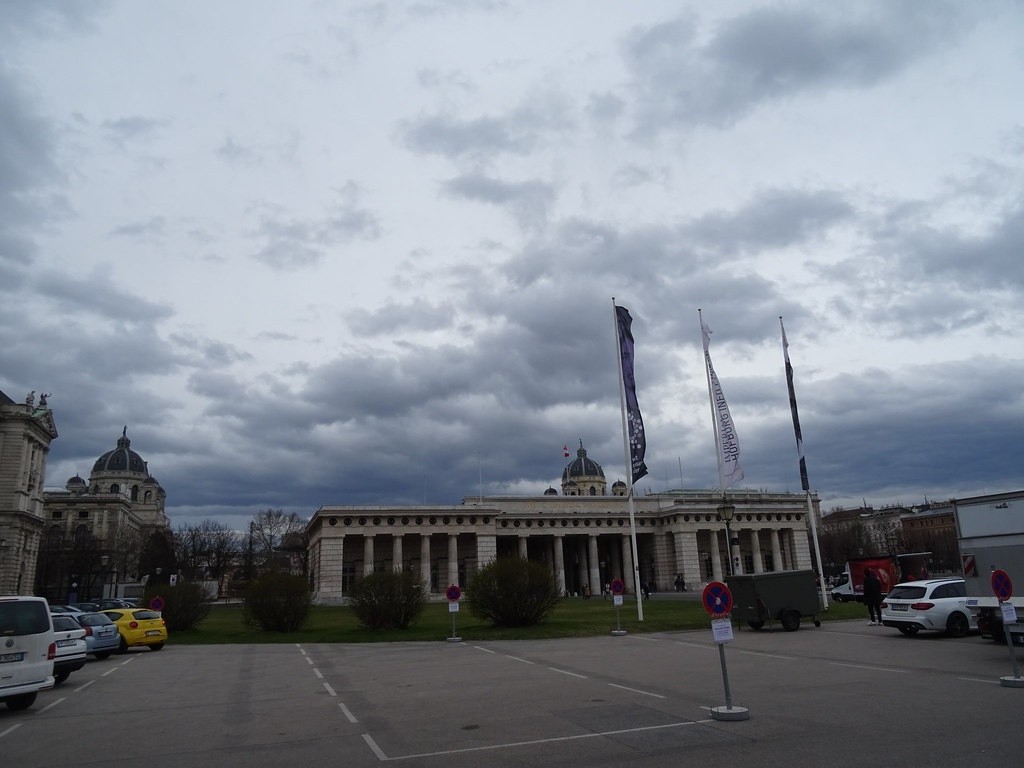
[881,576,982,640]
[50,617,88,685]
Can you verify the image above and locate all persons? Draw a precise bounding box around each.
[582,585,591,600]
[674,578,687,592]
[642,583,651,601]
[862,568,884,627]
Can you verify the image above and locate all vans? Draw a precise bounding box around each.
[0,596,58,711]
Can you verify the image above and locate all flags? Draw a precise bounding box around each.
[615,305,648,485]
[783,335,810,493]
[699,319,746,500]
[561,445,569,457]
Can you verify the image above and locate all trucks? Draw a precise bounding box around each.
[831,552,936,604]
[950,491,1024,644]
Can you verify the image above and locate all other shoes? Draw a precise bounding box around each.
[867,621,876,626]
[878,622,883,626]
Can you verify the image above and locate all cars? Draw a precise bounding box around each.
[101,608,168,654]
[50,597,145,617]
[66,611,121,659]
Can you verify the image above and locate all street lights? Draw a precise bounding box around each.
[716,492,735,576]
[99,555,111,598]
